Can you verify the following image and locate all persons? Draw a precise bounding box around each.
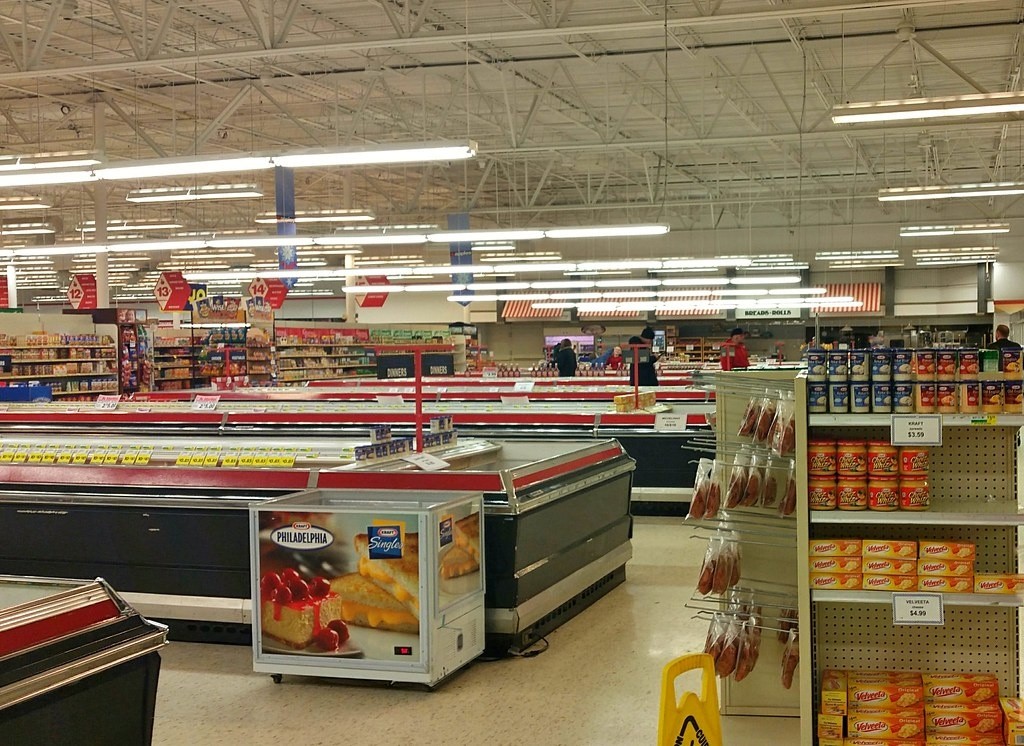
[554,343,559,368]
[123,310,135,323]
[721,328,749,371]
[986,325,1021,372]
[629,328,660,386]
[556,339,577,377]
[592,346,623,370]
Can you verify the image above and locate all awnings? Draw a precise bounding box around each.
[811,283,880,312]
[501,290,568,318]
[576,289,643,318]
[654,287,720,316]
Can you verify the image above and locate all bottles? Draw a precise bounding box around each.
[463,364,471,377]
[497,363,520,378]
[575,362,605,376]
[616,362,628,377]
[530,362,559,376]
[666,361,703,370]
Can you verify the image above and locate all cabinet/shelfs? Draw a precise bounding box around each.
[0,312,496,402]
[681,345,1024,746]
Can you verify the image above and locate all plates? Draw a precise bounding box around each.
[262,643,362,655]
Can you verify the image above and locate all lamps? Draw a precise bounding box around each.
[0,166,99,187]
[74,203,185,233]
[272,0,478,169]
[314,164,426,244]
[206,173,313,247]
[428,159,545,242]
[831,14,1024,123]
[877,66,1024,201]
[255,162,376,225]
[0,251,905,311]
[2,189,56,236]
[108,180,205,252]
[545,148,670,239]
[92,0,274,180]
[0,0,108,172]
[899,138,1009,236]
[912,245,999,266]
[126,59,264,202]
[0,182,52,212]
[13,185,107,256]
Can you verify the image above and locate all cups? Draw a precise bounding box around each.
[422,415,458,452]
[803,347,1024,416]
[352,424,414,467]
